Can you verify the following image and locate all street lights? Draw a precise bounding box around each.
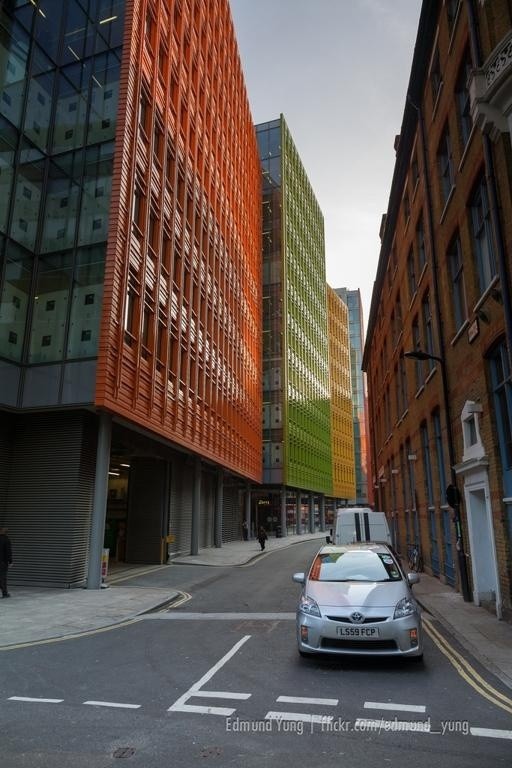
[404,350,472,606]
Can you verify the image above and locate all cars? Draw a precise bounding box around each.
[292,541,423,662]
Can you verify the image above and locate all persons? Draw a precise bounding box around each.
[241,518,249,541]
[256,526,268,551]
[0,526,12,597]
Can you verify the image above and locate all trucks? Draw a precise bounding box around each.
[327,506,392,550]
